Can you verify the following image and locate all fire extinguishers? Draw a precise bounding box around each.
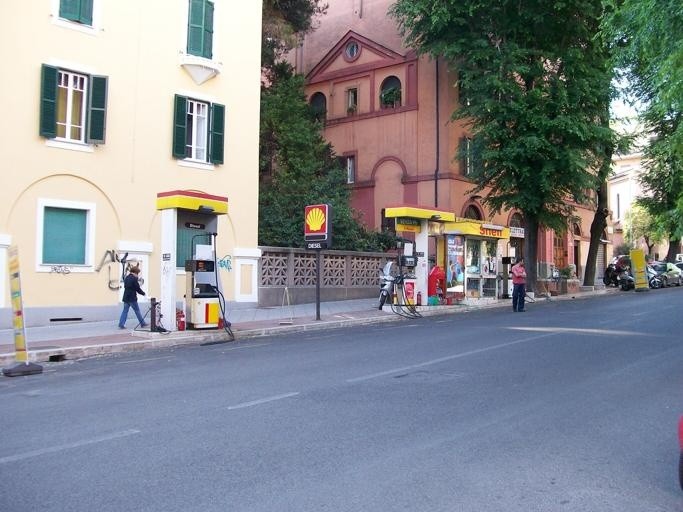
[177,310,185,331]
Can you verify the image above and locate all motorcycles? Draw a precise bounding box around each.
[620,265,662,291]
[603,264,627,287]
[374,267,408,310]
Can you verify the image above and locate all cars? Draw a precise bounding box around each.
[651,263,683,288]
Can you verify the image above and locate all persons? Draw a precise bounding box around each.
[118,266,149,330]
[449,260,459,288]
[512,256,527,312]
